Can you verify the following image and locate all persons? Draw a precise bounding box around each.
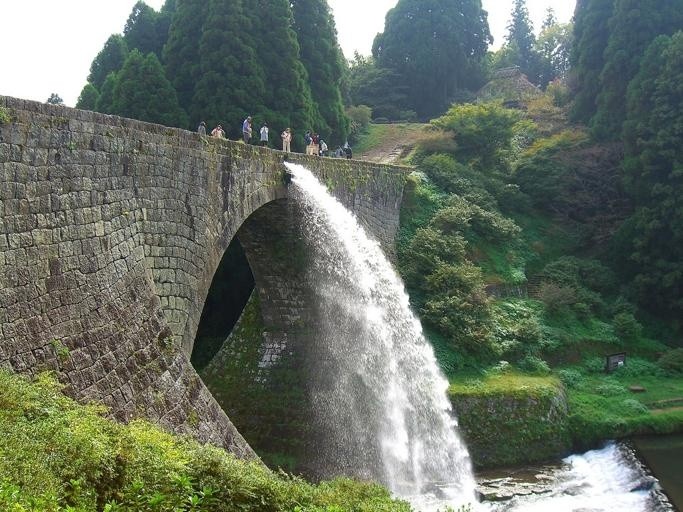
[211,125,225,137]
[280,127,292,152]
[344,144,353,159]
[198,121,207,135]
[304,130,329,156]
[335,145,343,158]
[242,116,253,144]
[259,122,269,146]
